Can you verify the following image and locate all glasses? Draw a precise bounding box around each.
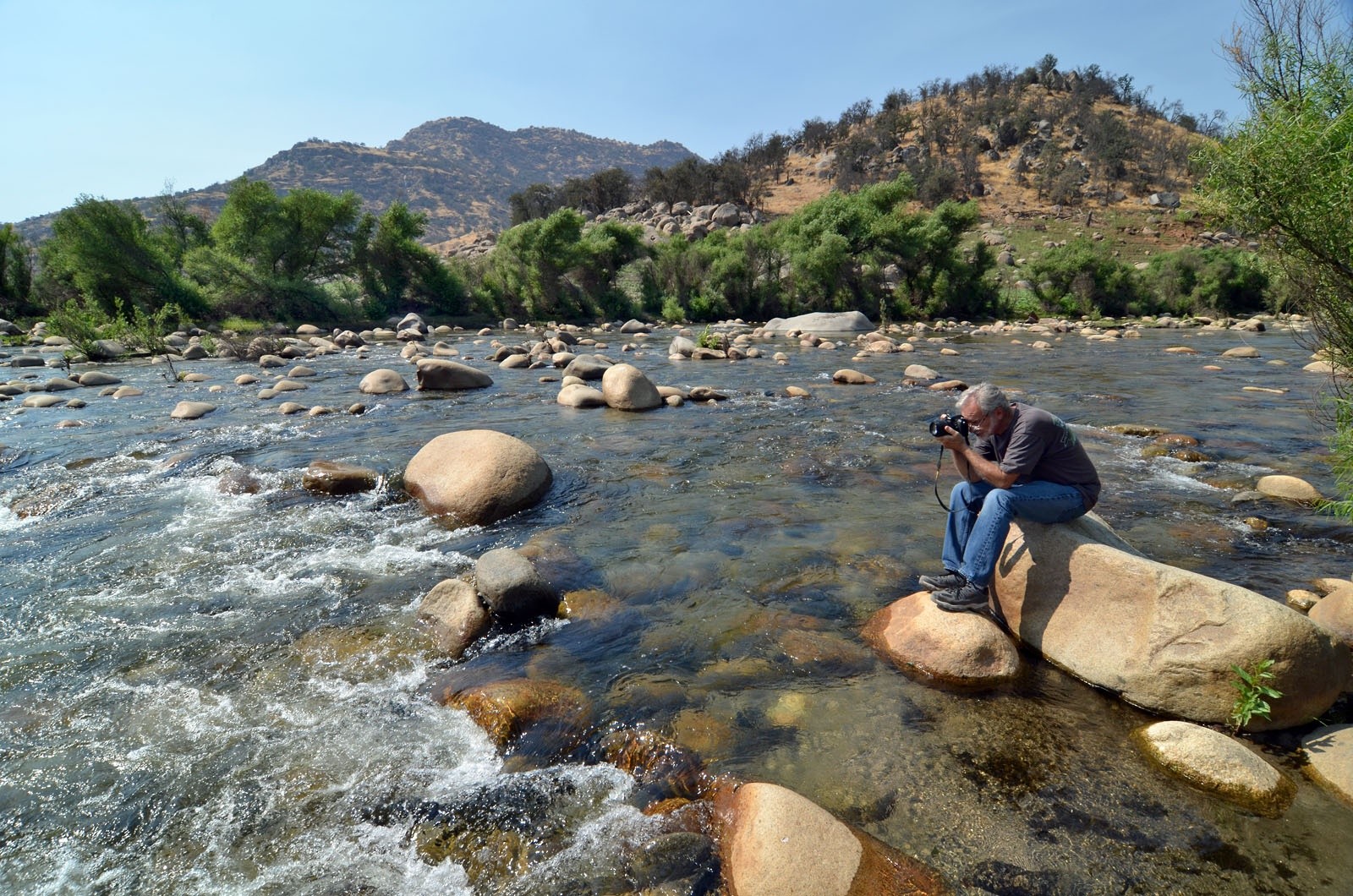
[968,411,990,430]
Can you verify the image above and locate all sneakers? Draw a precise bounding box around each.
[919,573,967,590]
[930,581,990,610]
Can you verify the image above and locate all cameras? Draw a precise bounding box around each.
[930,414,969,437]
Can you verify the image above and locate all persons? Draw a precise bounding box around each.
[919,383,1101,611]
